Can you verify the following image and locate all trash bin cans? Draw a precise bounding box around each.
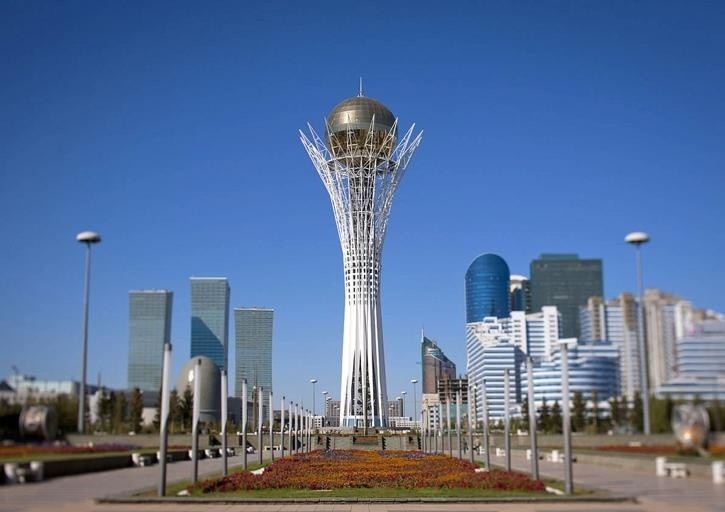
[712,460,725,484]
[496,448,500,456]
[204,449,209,457]
[4,461,17,483]
[132,452,140,466]
[552,450,559,462]
[656,456,668,476]
[30,460,43,480]
[188,450,192,459]
[526,449,531,460]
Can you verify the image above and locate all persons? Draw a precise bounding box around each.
[461,438,468,455]
[474,435,481,455]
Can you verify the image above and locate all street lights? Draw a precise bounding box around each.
[623,230,650,437]
[74,230,102,433]
[310,379,340,427]
[389,379,418,428]
